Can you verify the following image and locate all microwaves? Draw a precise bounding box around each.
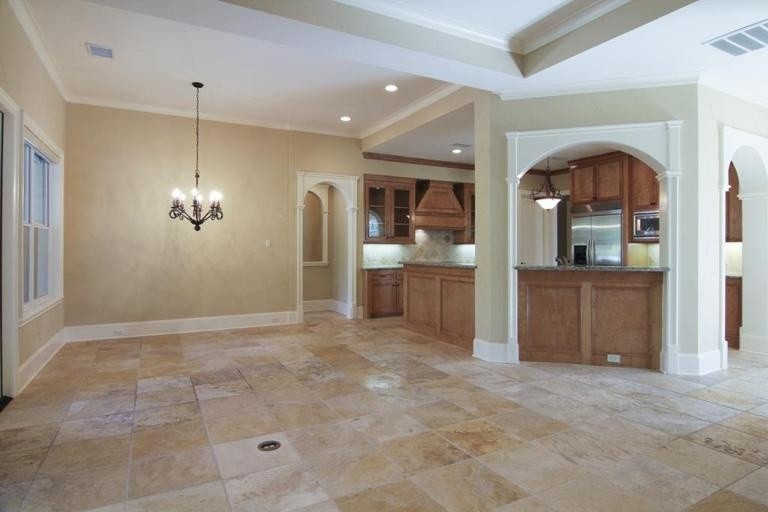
[633,209,660,241]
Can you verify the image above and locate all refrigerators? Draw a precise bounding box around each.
[571,208,622,266]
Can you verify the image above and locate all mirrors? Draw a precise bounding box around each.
[303,185,330,268]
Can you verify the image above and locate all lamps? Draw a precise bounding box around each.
[170,83,222,228]
[529,156,564,210]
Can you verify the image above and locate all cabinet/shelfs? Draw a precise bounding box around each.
[570,151,624,204]
[364,173,417,246]
[453,183,475,244]
[365,268,405,317]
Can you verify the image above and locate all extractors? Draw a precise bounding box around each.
[414,180,466,231]
